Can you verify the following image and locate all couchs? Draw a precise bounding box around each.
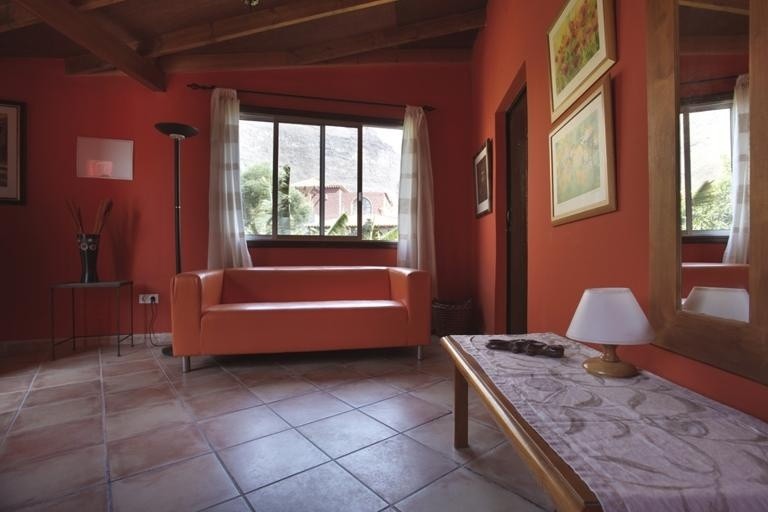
[169,266,433,373]
[680,261,750,298]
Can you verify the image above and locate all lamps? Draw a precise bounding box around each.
[682,287,749,325]
[154,122,199,357]
[240,0,264,11]
[564,287,656,378]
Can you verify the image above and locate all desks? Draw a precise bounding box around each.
[440,331,768,512]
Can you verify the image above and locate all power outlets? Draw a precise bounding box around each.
[139,294,159,304]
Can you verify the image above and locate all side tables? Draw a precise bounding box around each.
[49,280,133,361]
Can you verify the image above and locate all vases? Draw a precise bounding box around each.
[76,233,101,284]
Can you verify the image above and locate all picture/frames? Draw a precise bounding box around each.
[546,72,618,228]
[473,138,492,218]
[75,136,134,181]
[543,0,617,125]
[0,101,27,205]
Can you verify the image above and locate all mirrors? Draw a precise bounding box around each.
[642,0,768,388]
